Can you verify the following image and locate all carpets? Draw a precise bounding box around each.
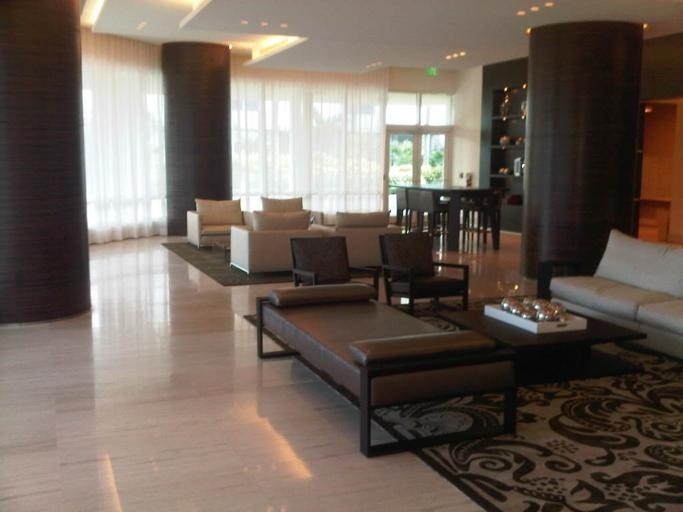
[161,243,384,287]
[244,294,683,511]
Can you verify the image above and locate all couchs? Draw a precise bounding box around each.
[186,210,324,250]
[549,227,682,358]
[230,225,402,276]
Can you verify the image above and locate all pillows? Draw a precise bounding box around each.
[195,199,251,225]
[252,211,310,228]
[260,196,304,210]
[336,210,390,227]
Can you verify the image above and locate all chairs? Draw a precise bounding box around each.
[288,235,379,301]
[395,189,506,241]
[379,234,470,315]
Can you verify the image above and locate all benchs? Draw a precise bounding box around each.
[256,283,519,456]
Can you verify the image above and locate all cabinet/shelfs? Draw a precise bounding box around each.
[492,114,525,179]
